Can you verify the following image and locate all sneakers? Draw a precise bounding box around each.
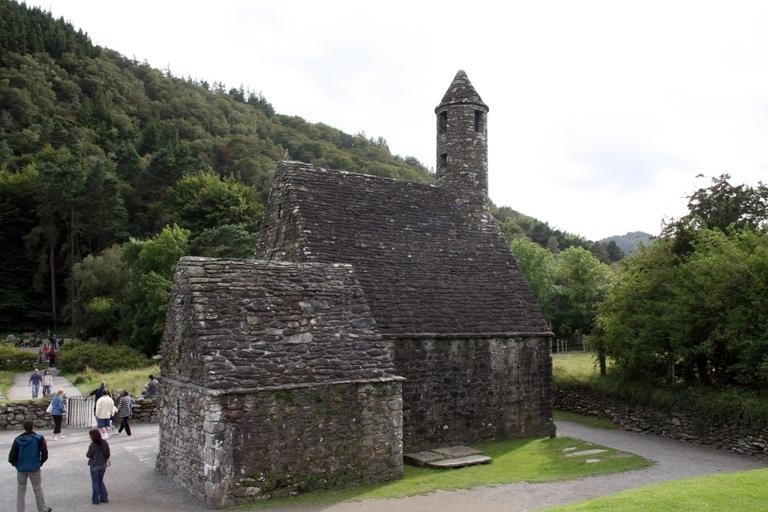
[100,426,131,438]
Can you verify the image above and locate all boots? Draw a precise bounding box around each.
[58,432,64,439]
[51,433,58,440]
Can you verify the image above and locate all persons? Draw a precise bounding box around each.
[7,421,53,512]
[95,390,114,441]
[51,390,64,434]
[86,428,110,505]
[83,381,108,435]
[15,328,75,367]
[113,390,136,437]
[41,369,53,397]
[28,367,43,399]
[141,374,159,399]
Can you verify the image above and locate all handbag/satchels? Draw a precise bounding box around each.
[45,401,53,413]
[105,458,112,467]
